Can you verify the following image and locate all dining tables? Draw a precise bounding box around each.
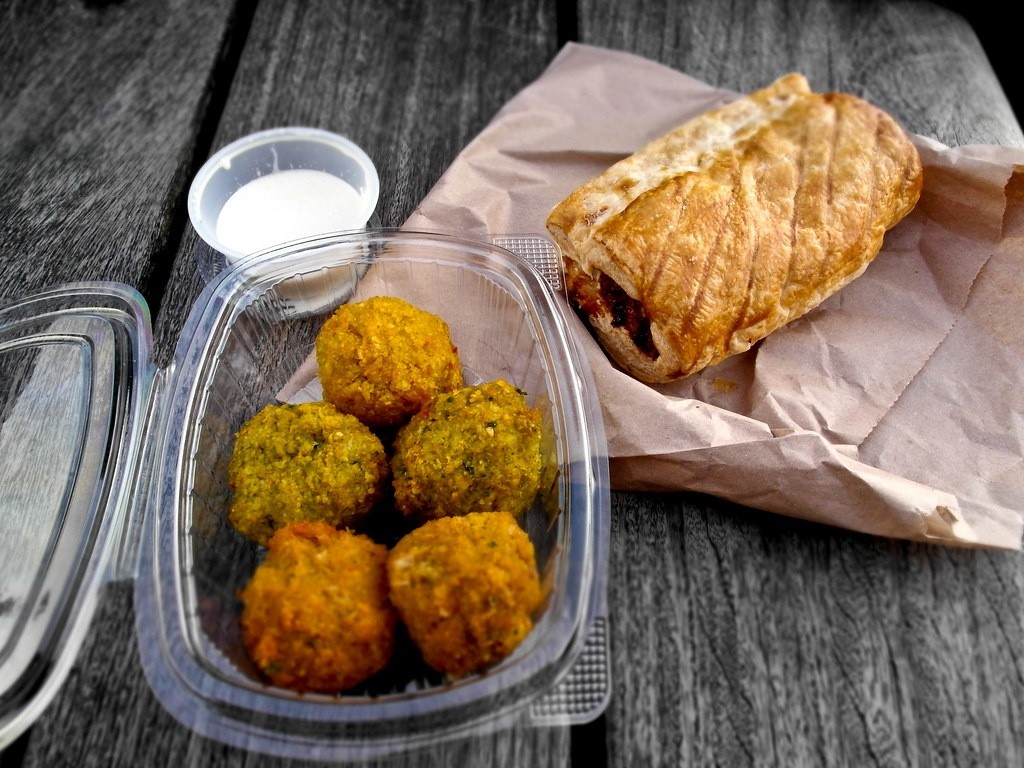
[0,1,1024,767]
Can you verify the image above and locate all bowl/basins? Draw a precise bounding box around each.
[184,127,383,297]
[1,225,616,767]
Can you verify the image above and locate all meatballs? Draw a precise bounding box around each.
[224,295,542,698]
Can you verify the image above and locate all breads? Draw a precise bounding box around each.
[548,72,918,379]
